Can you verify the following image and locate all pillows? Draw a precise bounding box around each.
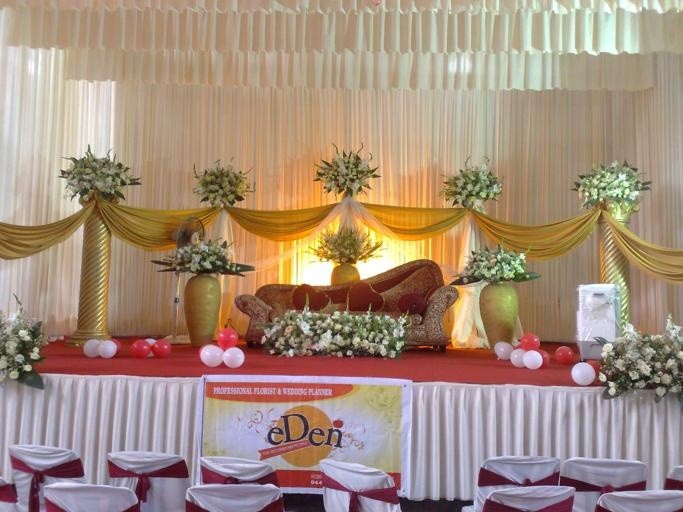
[346,280,385,312]
[399,294,427,313]
[291,284,331,311]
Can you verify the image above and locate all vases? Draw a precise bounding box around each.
[596,204,639,338]
[184,273,221,347]
[331,262,360,286]
[479,281,520,352]
[67,197,118,347]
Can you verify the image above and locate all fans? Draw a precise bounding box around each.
[164,217,205,344]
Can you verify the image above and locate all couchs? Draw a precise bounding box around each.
[235,259,459,352]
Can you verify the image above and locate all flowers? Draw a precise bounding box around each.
[149,236,256,277]
[451,242,542,282]
[438,155,505,215]
[571,160,652,207]
[305,224,382,263]
[599,313,683,403]
[0,293,49,389]
[193,156,255,208]
[58,144,141,202]
[311,142,382,197]
[256,304,409,359]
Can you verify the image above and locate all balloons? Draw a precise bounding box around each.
[215,327,238,350]
[493,332,549,369]
[222,347,245,369]
[199,344,223,368]
[554,345,574,366]
[570,362,596,386]
[81,336,173,360]
